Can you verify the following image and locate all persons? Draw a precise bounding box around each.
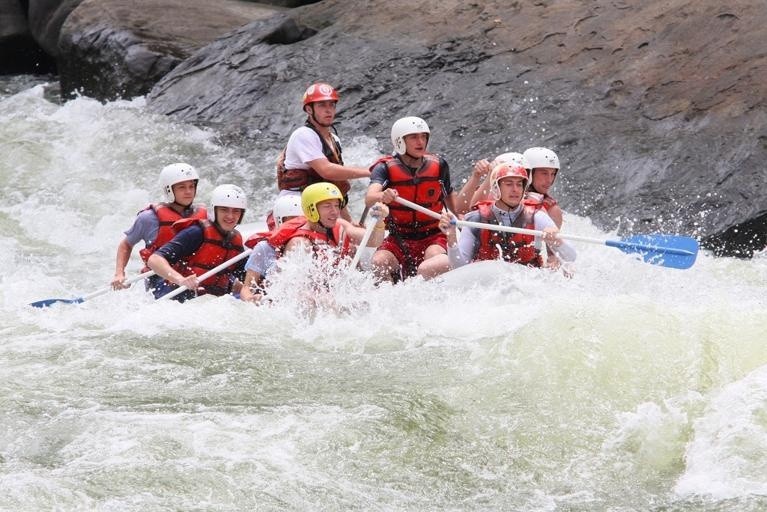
[273,82,371,229]
[522,147,563,232]
[364,116,458,285]
[110,164,207,292]
[239,192,306,308]
[147,183,250,302]
[418,152,523,282]
[438,162,564,270]
[281,182,389,291]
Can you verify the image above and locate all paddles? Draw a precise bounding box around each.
[32,270,155,308]
[450,221,699,269]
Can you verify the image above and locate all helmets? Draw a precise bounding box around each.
[301,82,340,108]
[523,146,561,171]
[390,116,432,156]
[207,183,248,226]
[490,152,523,167]
[489,163,530,202]
[158,161,199,205]
[271,193,306,230]
[302,180,347,224]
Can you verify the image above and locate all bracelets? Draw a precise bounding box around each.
[372,224,387,232]
